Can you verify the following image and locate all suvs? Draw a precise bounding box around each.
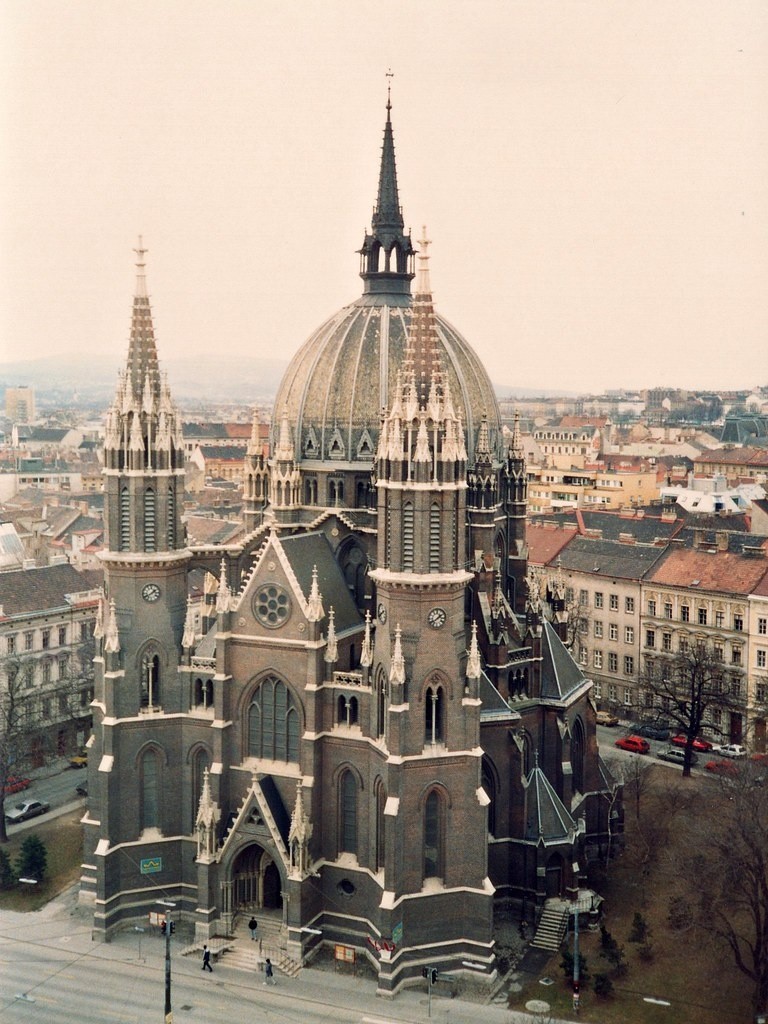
[704,758,741,778]
[711,743,748,760]
[657,748,698,765]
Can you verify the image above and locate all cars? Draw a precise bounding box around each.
[671,733,713,753]
[630,722,669,741]
[76,779,88,796]
[66,747,88,769]
[595,711,619,727]
[5,797,51,822]
[5,776,30,794]
[752,753,768,767]
[615,735,651,754]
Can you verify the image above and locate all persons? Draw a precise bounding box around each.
[263,958,276,985]
[202,945,213,972]
[249,916,257,941]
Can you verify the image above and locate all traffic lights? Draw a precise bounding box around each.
[430,969,439,985]
[422,967,428,978]
[161,920,166,935]
[171,921,176,935]
